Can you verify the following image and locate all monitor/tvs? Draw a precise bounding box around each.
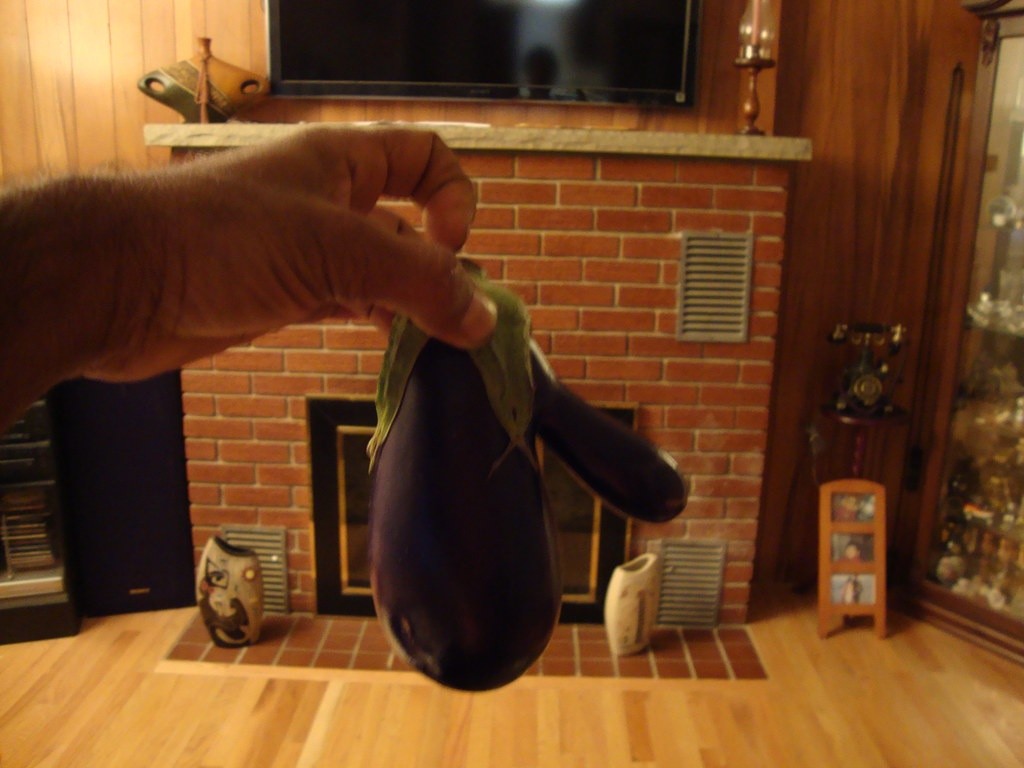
[267,0,699,111]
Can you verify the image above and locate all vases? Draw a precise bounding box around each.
[603,553,661,658]
[197,537,262,647]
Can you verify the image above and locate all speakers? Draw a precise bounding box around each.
[44,366,197,618]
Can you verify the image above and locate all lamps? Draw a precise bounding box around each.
[735,0,776,133]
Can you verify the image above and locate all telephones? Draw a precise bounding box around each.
[826,321,911,414]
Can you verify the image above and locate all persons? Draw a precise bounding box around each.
[0,126,496,427]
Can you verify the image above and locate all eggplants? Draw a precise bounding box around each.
[365,255,562,691]
[531,341,689,521]
